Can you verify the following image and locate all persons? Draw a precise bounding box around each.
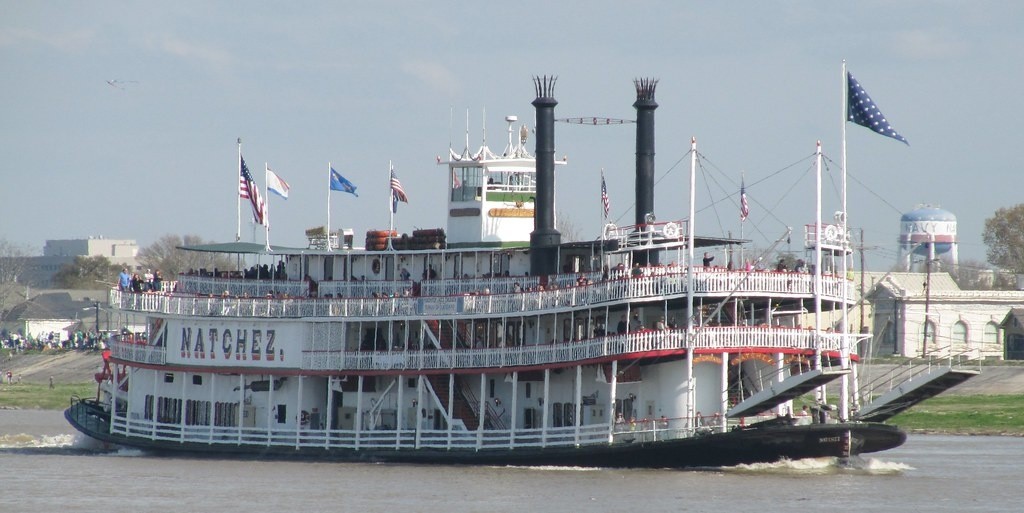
[422,264,437,280]
[727,256,815,274]
[602,261,688,294]
[188,260,285,278]
[49,376,55,390]
[614,411,719,437]
[8,325,146,353]
[617,314,631,335]
[462,275,593,315]
[400,268,410,280]
[636,315,678,334]
[801,405,808,426]
[703,251,715,271]
[209,289,410,318]
[118,268,163,292]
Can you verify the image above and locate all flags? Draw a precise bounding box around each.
[454,172,461,189]
[239,155,267,227]
[846,72,912,147]
[266,168,290,201]
[601,176,609,219]
[330,167,358,196]
[740,186,749,221]
[390,169,408,214]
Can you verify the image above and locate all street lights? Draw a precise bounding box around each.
[912,259,931,358]
[83,307,110,332]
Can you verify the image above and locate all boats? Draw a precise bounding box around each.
[60,70,981,465]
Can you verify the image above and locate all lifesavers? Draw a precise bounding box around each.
[663,222,679,239]
[824,225,837,240]
[365,230,397,251]
